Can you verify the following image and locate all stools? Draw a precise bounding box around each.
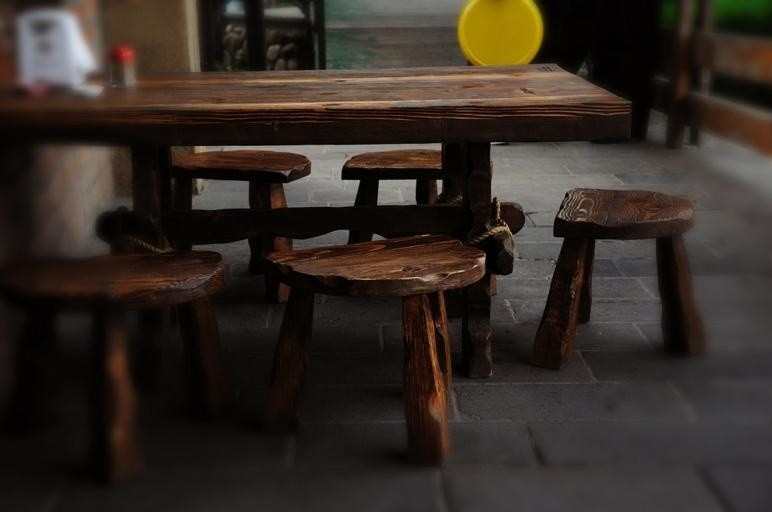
[2,251,235,490]
[342,150,443,245]
[171,150,310,304]
[260,234,487,467]
[526,187,706,370]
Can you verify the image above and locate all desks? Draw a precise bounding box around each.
[0,63,632,378]
